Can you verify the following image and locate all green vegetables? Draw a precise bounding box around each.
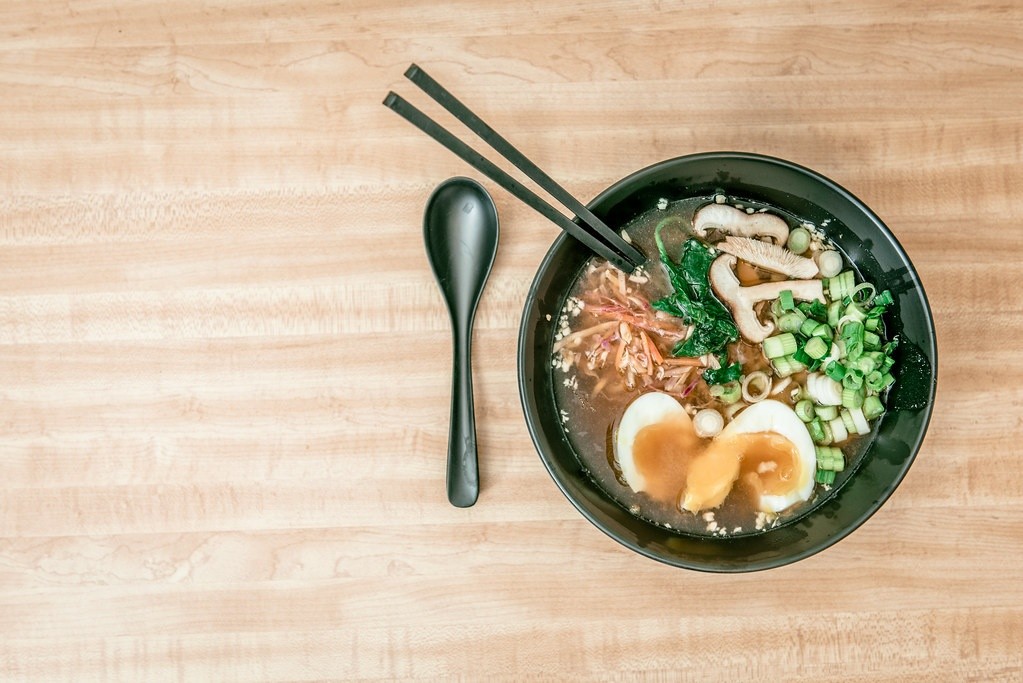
[648,214,743,385]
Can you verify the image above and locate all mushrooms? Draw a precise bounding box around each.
[691,202,826,343]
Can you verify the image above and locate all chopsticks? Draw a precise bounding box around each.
[382,62,647,277]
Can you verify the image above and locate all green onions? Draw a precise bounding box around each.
[693,227,898,485]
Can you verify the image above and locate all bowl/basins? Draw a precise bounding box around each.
[519,151,940,573]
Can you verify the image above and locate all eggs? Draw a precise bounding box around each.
[615,389,815,512]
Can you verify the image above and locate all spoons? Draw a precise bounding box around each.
[423,175,499,509]
